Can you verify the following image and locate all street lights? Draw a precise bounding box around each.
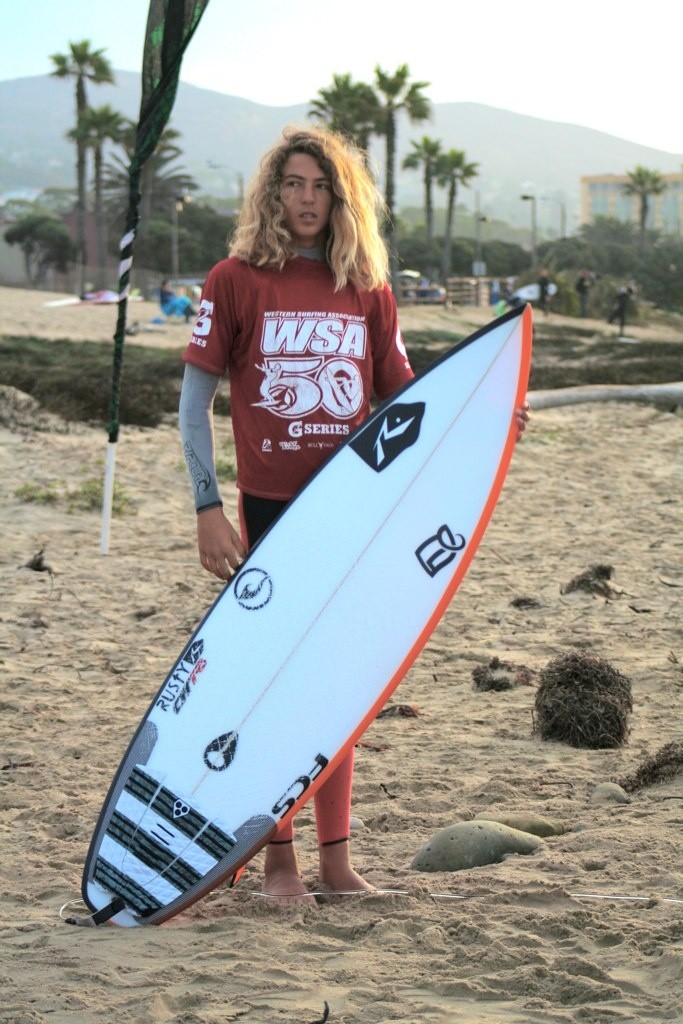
[522,194,540,277]
[172,194,191,278]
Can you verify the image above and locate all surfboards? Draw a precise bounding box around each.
[83,298,533,926]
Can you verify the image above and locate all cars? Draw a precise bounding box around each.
[397,268,447,306]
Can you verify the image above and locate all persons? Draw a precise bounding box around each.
[179,129,531,910]
[539,268,549,317]
[577,269,589,317]
[609,288,634,334]
[160,279,198,322]
